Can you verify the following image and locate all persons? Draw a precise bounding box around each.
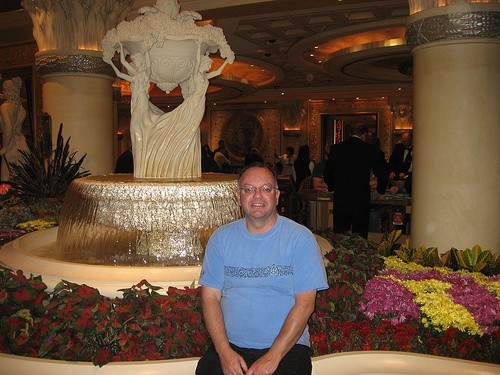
[196,164,330,375]
[199,138,326,226]
[0,76,33,184]
[102,42,228,179]
[323,122,388,241]
[371,137,380,147]
[114,141,133,173]
[365,132,374,143]
[387,131,413,182]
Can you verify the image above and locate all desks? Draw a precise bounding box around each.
[296,191,411,234]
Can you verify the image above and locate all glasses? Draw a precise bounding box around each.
[239,184,276,193]
